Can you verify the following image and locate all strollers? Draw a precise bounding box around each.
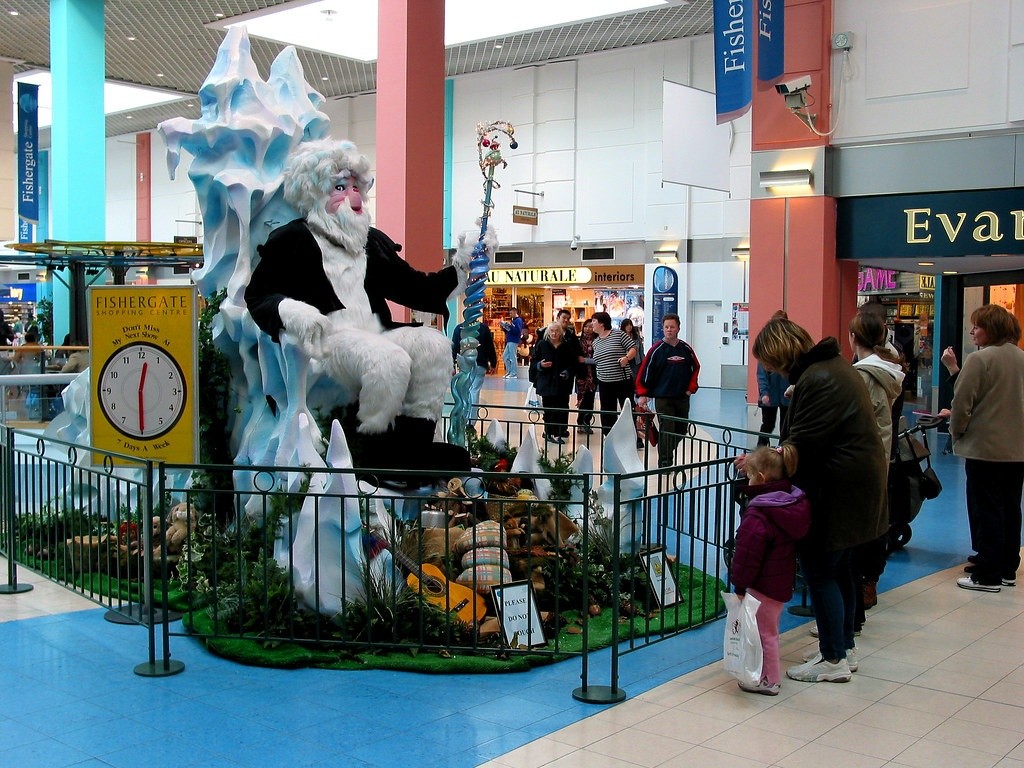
[883,417,942,549]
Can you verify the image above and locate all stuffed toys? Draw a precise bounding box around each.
[503,514,525,549]
[131,501,199,557]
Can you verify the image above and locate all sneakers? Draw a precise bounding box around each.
[956,576,1002,593]
[802,649,858,672]
[786,653,852,683]
[1002,577,1016,586]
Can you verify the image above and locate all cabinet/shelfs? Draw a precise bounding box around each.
[0,302,34,328]
[483,293,512,323]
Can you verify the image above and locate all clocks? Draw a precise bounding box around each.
[96,340,186,441]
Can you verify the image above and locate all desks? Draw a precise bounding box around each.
[45,370,79,421]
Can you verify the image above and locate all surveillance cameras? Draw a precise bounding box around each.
[570,240,578,250]
[774,74,812,94]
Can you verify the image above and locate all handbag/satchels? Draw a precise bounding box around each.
[922,468,942,499]
[720,591,764,688]
[524,382,545,413]
[635,400,660,447]
[616,361,633,398]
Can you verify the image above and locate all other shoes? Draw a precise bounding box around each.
[559,429,569,437]
[502,372,510,378]
[542,433,565,444]
[809,626,862,638]
[577,426,593,434]
[861,620,866,625]
[506,374,518,378]
[636,442,644,451]
[659,463,671,474]
[466,425,476,432]
[738,675,779,696]
[863,581,877,610]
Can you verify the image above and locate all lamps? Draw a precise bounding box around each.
[759,169,812,187]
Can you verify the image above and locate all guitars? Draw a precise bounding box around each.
[362,524,488,625]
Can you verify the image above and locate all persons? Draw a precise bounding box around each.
[756,310,794,447]
[732,318,889,684]
[450,308,497,433]
[0,310,88,372]
[500,308,523,379]
[244,139,498,478]
[529,310,645,443]
[635,314,701,476]
[847,301,907,488]
[732,445,811,696]
[937,304,1024,593]
[517,318,529,367]
